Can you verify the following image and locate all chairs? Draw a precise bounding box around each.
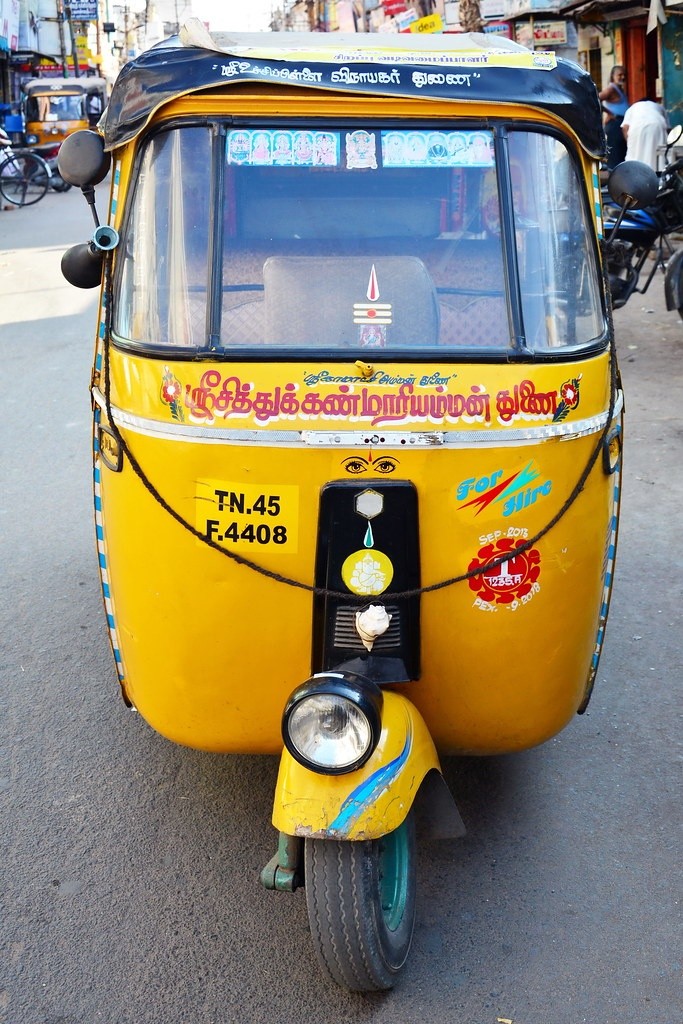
[261,255,440,348]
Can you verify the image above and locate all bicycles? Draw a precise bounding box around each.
[0,127,72,209]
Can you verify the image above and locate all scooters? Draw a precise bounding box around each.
[9,142,72,193]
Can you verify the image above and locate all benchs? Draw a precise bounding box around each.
[183,236,542,351]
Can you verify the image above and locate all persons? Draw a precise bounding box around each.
[598,66,628,169]
[621,97,676,187]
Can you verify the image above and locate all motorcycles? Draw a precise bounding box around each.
[602,124,682,321]
[61,25,627,994]
[19,77,107,148]
